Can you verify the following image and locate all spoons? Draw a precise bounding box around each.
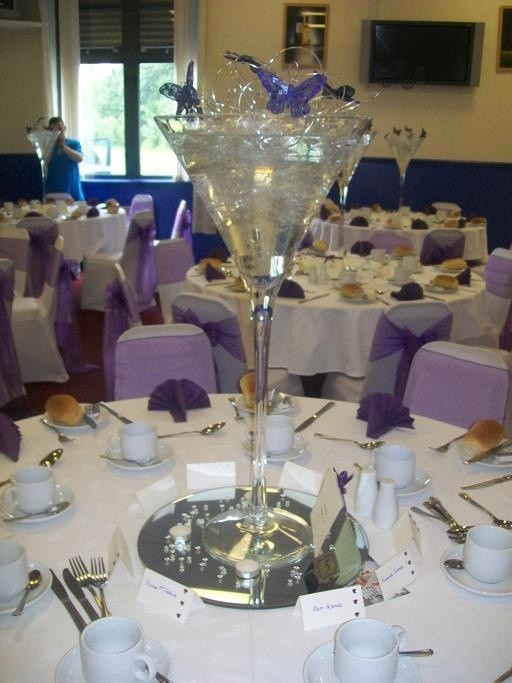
[444,557,464,570]
[157,420,228,439]
[397,646,433,657]
[310,429,385,449]
[410,497,474,542]
[98,453,162,467]
[0,446,64,482]
[144,662,172,683]
[2,499,69,522]
[12,569,43,617]
[459,491,512,528]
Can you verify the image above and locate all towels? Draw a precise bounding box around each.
[356,393,415,440]
[147,379,212,422]
[204,263,226,282]
[391,282,424,301]
[278,280,305,299]
[319,204,333,220]
[411,218,427,229]
[420,250,442,266]
[350,216,368,226]
[0,413,21,461]
[457,268,471,286]
[350,241,375,256]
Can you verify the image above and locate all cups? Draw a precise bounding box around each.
[335,617,407,682]
[359,461,434,495]
[4,466,55,513]
[78,615,156,680]
[0,541,30,598]
[107,421,159,461]
[370,443,417,488]
[53,633,170,682]
[337,271,357,292]
[245,414,294,454]
[52,198,66,207]
[401,254,424,264]
[355,468,377,517]
[375,478,399,528]
[371,247,389,264]
[0,480,76,522]
[465,523,511,581]
[78,200,88,212]
[28,198,38,208]
[394,266,412,281]
[302,634,419,683]
[2,200,13,210]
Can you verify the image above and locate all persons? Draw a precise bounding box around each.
[27,118,84,202]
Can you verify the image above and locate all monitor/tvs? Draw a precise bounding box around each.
[360,19,484,87]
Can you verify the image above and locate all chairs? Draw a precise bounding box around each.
[0,221,30,301]
[149,237,195,323]
[0,258,23,409]
[12,234,71,385]
[431,203,464,218]
[79,210,153,308]
[129,194,155,212]
[171,290,248,395]
[113,322,218,400]
[361,301,456,402]
[44,192,75,207]
[112,261,144,392]
[13,216,60,300]
[169,199,190,242]
[399,339,512,442]
[419,227,468,264]
[484,247,512,335]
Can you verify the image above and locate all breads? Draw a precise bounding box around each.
[340,282,362,299]
[472,217,486,226]
[311,239,330,252]
[444,219,461,229]
[433,274,459,289]
[239,372,269,407]
[198,257,221,273]
[329,213,344,222]
[234,276,246,289]
[392,243,412,256]
[324,204,339,213]
[46,395,83,425]
[463,417,502,460]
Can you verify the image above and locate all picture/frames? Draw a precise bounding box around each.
[280,2,330,71]
[495,5,512,71]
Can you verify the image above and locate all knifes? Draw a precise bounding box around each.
[99,400,133,423]
[460,473,512,490]
[294,401,338,435]
[464,436,512,464]
[61,565,101,628]
[42,566,86,634]
[299,292,332,303]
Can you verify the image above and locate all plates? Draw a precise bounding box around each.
[241,433,312,460]
[441,541,511,595]
[424,281,459,293]
[458,433,512,468]
[42,403,103,430]
[0,556,53,612]
[105,438,176,469]
[388,276,416,286]
[338,283,377,304]
[236,390,296,415]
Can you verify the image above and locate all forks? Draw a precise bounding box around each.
[228,395,245,422]
[38,417,77,443]
[428,429,482,454]
[67,553,113,621]
[87,554,109,619]
